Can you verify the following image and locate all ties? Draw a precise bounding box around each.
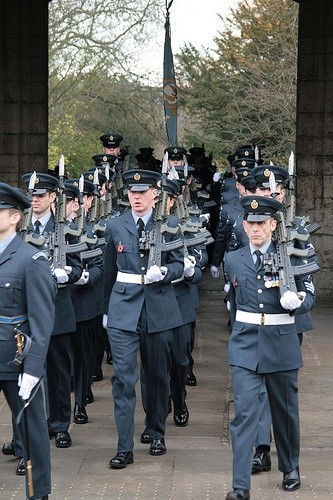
[137,218,145,242]
[254,250,261,269]
[34,220,41,235]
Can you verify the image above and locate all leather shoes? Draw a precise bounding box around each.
[251,452,271,471]
[46,352,198,468]
[282,466,300,491]
[225,488,250,500]
[1,443,14,456]
[17,457,26,475]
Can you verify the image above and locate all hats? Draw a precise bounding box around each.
[100,134,123,148]
[227,144,289,222]
[22,153,116,198]
[121,145,205,198]
[0,183,31,211]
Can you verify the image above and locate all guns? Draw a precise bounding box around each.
[284,150,322,242]
[263,170,320,300]
[138,143,217,270]
[18,142,134,290]
[252,146,275,168]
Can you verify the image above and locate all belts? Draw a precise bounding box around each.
[116,271,184,284]
[235,310,295,326]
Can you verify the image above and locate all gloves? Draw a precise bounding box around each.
[103,314,109,329]
[183,255,196,277]
[74,269,90,285]
[54,266,72,283]
[210,265,220,278]
[147,265,167,282]
[280,290,306,310]
[18,373,39,400]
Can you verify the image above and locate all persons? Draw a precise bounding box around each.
[0,134,316,500]
